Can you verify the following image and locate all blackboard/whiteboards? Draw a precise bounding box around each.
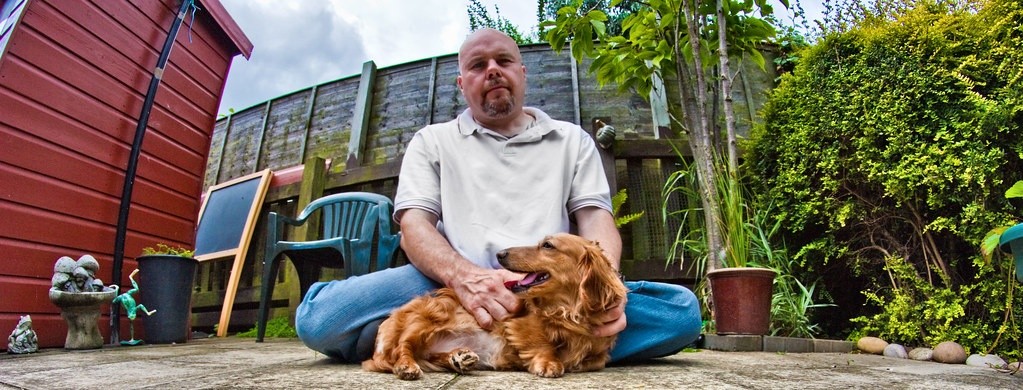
[191,165,274,263]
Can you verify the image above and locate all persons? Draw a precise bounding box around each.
[295,28,701,368]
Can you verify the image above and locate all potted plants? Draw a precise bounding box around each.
[135,243,200,344]
[979,180,1023,284]
[658,132,777,335]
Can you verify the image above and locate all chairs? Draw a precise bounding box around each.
[256,191,402,343]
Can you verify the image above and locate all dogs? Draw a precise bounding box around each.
[361,232,630,381]
